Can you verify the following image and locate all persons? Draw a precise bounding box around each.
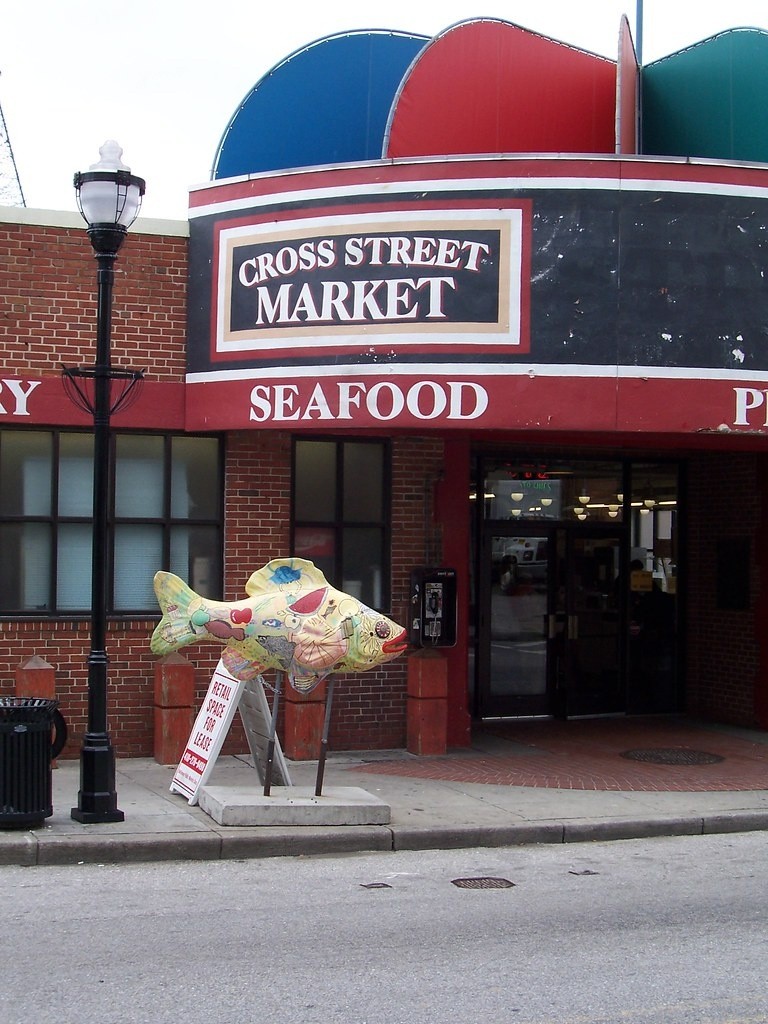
[633,580,677,673]
[611,560,644,625]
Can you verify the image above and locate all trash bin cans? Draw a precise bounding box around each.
[0,696,67,831]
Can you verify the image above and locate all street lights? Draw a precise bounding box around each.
[55,136,147,821]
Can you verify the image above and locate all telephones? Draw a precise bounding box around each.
[425,582,443,619]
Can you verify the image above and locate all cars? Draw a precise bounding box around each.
[492,535,550,597]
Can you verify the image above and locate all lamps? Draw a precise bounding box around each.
[470,483,661,522]
